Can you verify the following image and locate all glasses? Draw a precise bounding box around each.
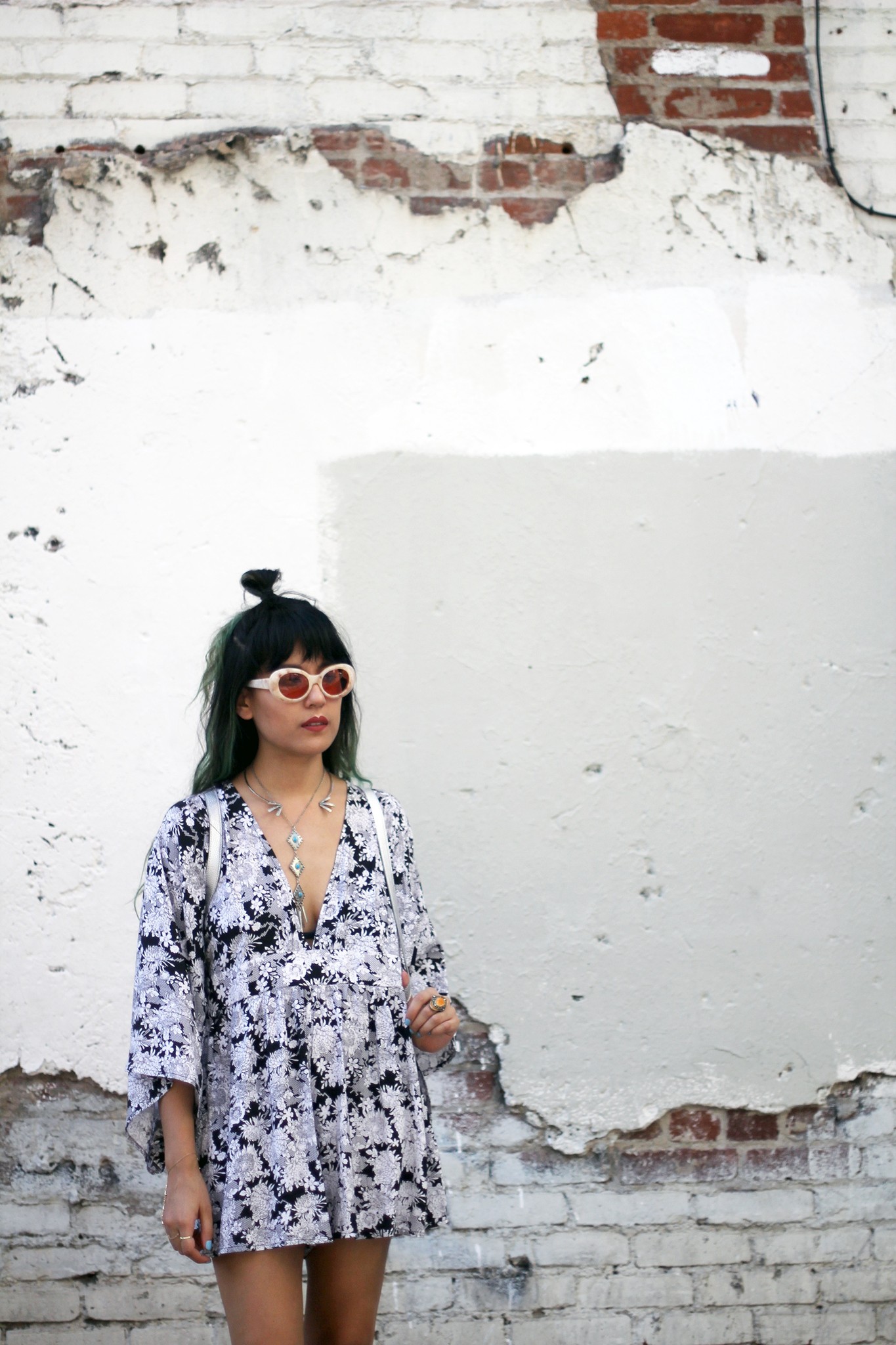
[249,664,355,703]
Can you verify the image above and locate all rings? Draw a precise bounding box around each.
[178,1236,194,1240]
[428,995,447,1011]
[167,1233,179,1240]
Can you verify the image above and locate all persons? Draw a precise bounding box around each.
[124,565,461,1345]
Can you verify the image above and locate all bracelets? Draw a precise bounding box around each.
[160,1152,197,1226]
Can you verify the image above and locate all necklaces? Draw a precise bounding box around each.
[242,760,334,932]
